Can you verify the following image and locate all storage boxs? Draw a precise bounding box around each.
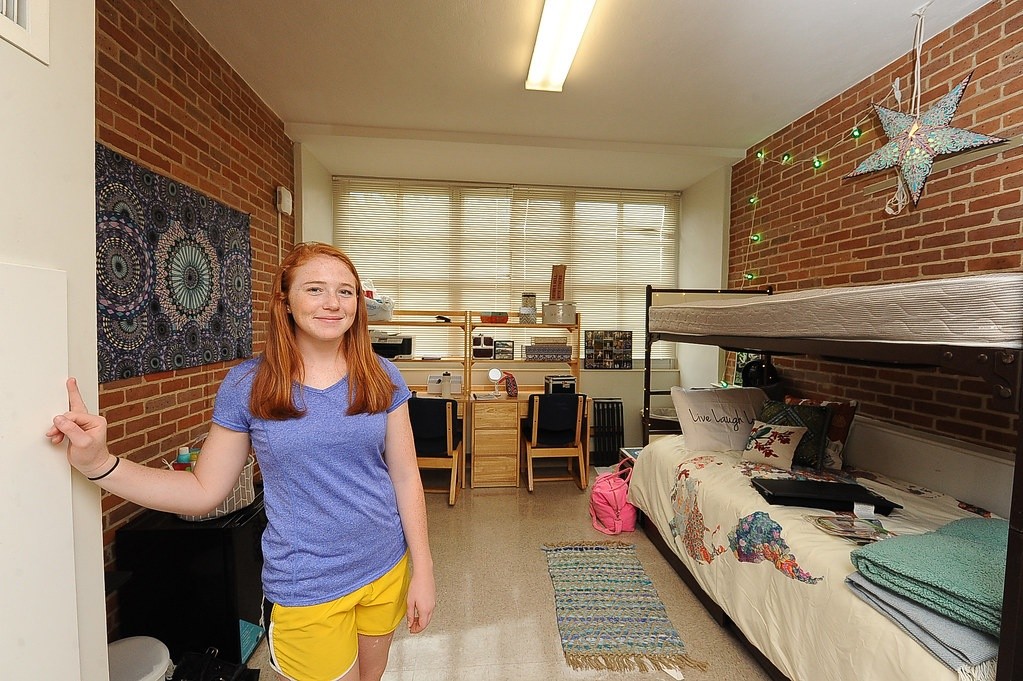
[428,375,462,394]
[179,455,255,522]
[619,447,643,480]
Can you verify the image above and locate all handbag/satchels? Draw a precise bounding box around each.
[499,371,518,397]
[588,457,638,535]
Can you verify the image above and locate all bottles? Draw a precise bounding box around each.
[177,447,190,463]
[190,448,201,470]
[442,372,451,396]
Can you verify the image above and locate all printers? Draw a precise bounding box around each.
[369,331,415,361]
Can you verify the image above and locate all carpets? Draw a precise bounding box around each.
[544,540,706,670]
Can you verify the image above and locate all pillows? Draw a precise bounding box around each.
[741,420,808,471]
[761,399,832,474]
[785,395,858,470]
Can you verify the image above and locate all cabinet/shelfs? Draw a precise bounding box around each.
[473,401,519,491]
[366,310,583,395]
[112,486,272,665]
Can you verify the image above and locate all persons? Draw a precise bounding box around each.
[47,241,437,681]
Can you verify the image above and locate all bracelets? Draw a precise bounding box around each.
[87,457,119,480]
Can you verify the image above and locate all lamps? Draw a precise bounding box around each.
[525,0,597,92]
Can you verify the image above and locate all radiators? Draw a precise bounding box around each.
[592,397,625,466]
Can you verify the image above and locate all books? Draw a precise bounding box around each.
[844,536,870,545]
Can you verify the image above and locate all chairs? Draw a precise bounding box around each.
[671,385,768,451]
[521,394,587,491]
[408,398,464,505]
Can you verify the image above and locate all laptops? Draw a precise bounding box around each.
[749,478,903,516]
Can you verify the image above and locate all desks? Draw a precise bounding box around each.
[408,392,468,490]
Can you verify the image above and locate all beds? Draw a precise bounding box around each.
[641,272,1023,681]
